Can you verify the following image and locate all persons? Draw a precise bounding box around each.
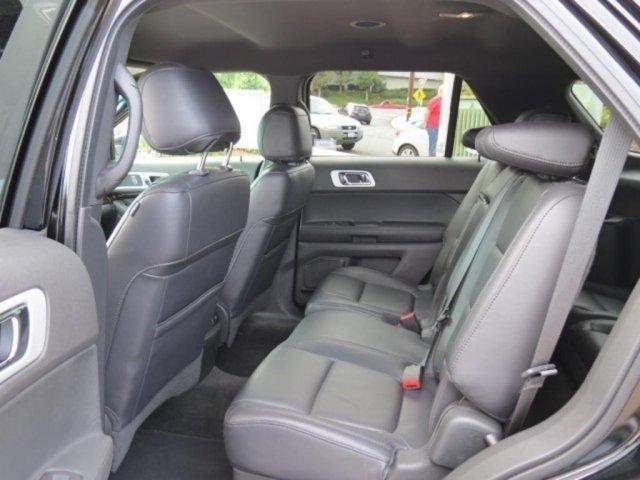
[418,80,444,157]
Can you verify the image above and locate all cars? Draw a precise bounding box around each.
[393,120,429,157]
[310,95,363,150]
[339,102,371,125]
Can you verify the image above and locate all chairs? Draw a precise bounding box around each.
[100,58,596,480]
